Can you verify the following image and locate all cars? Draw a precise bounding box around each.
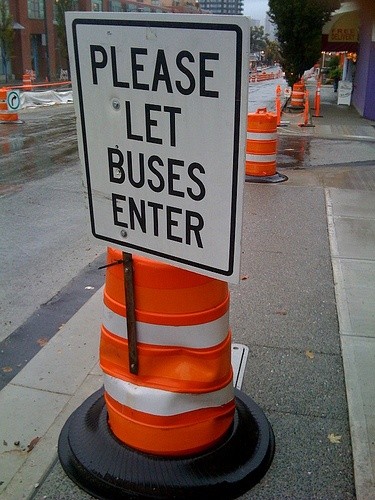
[282,72,293,79]
[257,67,262,72]
